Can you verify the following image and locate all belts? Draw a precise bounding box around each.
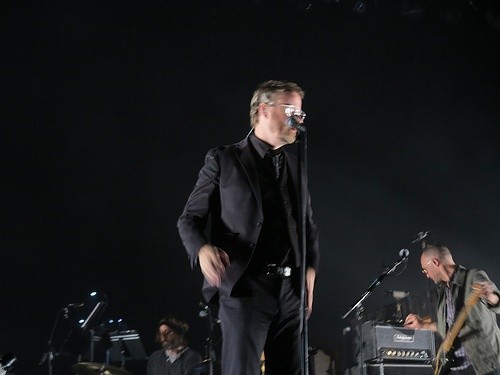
[263,266,298,278]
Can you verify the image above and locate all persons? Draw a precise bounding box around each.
[146,317,204,375]
[176,80,321,374]
[403,242,500,375]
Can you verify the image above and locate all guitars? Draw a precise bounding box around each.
[433,282,488,374]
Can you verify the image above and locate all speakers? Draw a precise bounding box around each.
[362,362,433,375]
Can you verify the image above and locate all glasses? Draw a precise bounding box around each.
[266,102,306,120]
[422,260,434,274]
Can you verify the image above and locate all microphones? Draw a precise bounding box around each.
[285,116,307,133]
[384,289,410,299]
[399,248,411,261]
[79,299,107,331]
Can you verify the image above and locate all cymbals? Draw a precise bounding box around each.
[69,362,130,375]
